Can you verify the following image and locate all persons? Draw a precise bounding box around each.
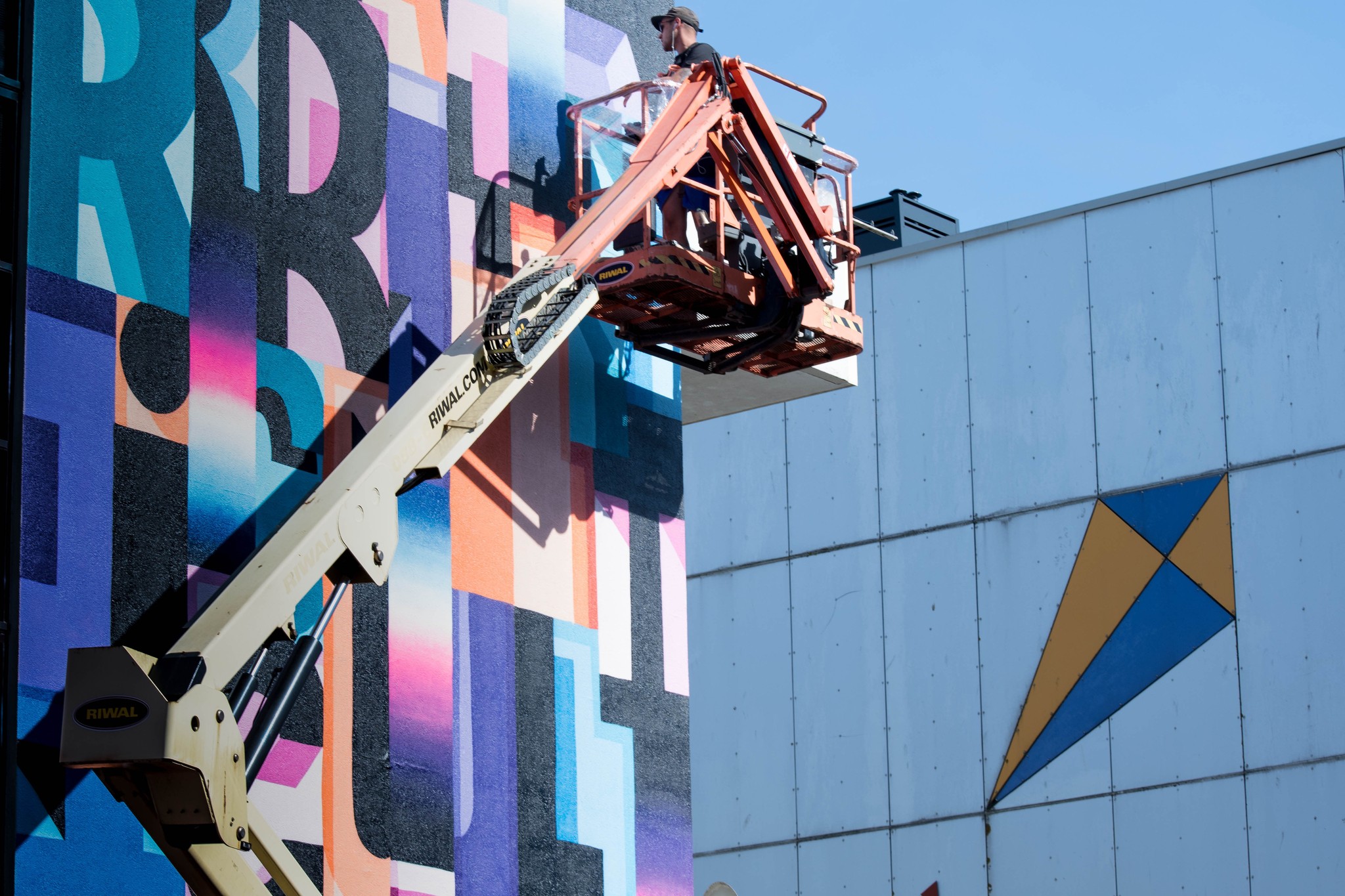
[651,6,732,253]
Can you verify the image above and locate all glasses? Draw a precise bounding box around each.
[658,19,683,32]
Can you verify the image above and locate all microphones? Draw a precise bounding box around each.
[673,23,678,29]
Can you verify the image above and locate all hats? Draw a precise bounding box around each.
[651,7,703,33]
